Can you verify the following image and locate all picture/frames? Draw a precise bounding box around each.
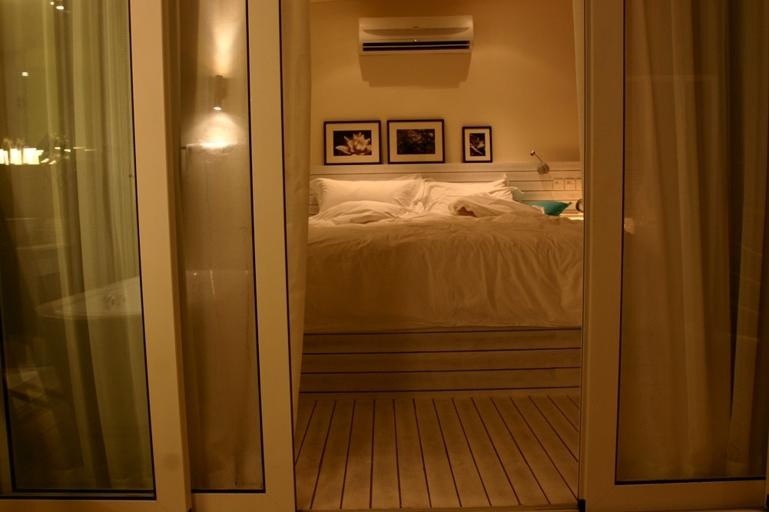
[322,117,494,165]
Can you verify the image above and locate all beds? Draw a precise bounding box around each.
[306,164,583,325]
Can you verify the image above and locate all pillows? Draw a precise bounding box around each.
[309,176,571,219]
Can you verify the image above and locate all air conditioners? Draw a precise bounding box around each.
[355,14,473,52]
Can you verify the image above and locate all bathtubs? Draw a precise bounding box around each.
[37,267,252,482]
